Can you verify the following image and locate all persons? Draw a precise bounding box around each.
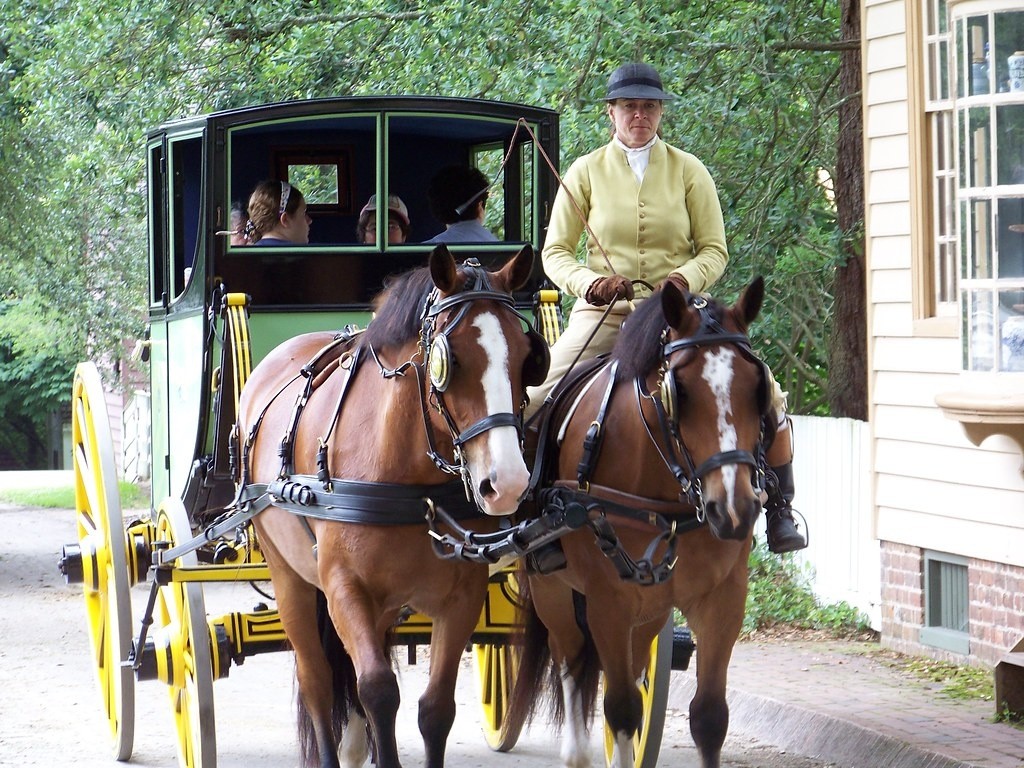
[229,180,313,246]
[356,193,411,244]
[522,60,806,555]
[419,163,500,245]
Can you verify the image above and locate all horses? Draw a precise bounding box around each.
[237,244,535,768]
[505,275,765,768]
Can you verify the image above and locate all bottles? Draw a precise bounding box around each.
[1007,51,1024,93]
[1002,316,1024,372]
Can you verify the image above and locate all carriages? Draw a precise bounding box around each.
[57,94,776,768]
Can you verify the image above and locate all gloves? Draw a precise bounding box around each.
[652,274,689,295]
[585,275,634,306]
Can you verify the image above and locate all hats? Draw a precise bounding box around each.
[598,63,674,100]
[359,192,410,227]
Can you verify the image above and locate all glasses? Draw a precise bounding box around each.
[363,223,401,236]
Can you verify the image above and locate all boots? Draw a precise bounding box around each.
[519,498,567,573]
[763,455,805,551]
